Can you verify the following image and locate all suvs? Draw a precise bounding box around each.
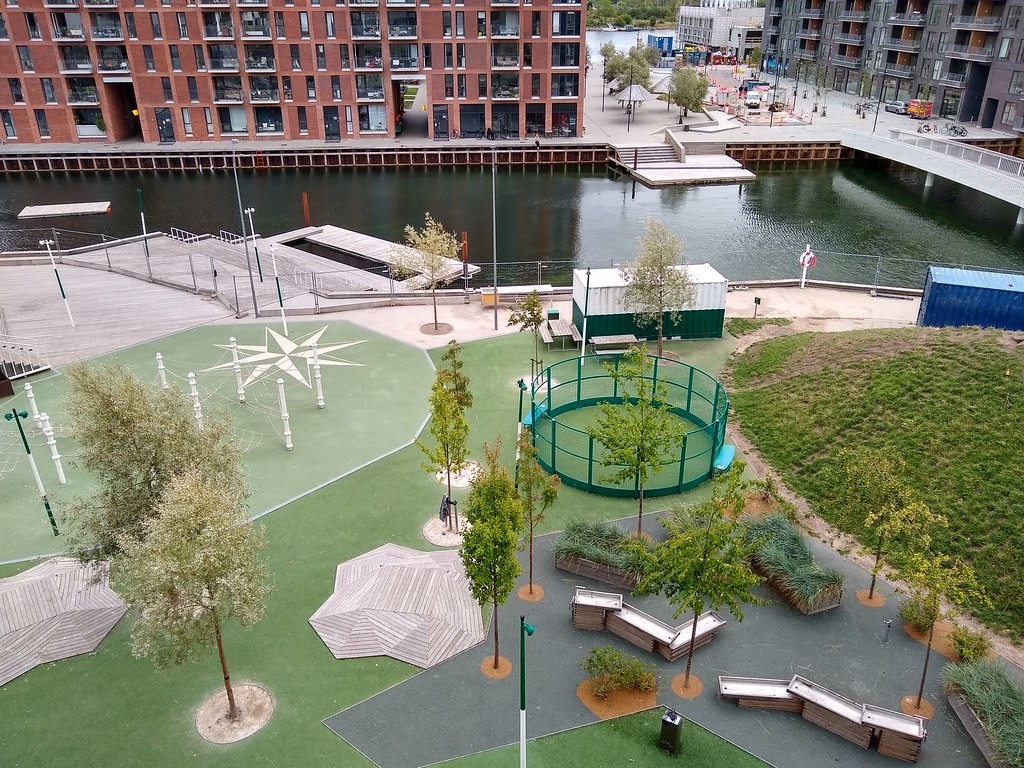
[884,101,908,114]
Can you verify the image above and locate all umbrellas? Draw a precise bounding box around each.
[649,76,677,111]
[605,74,624,108]
[615,84,654,121]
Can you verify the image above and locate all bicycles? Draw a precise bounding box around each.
[854,103,877,114]
[451,129,467,139]
[917,121,968,137]
[476,128,506,139]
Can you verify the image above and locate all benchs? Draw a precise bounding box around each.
[523,404,547,432]
[589,338,646,351]
[713,445,735,478]
[569,325,583,350]
[538,325,554,351]
[595,349,632,364]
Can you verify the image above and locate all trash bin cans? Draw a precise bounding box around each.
[610,88,613,94]
[658,710,683,755]
[547,309,559,330]
[685,125,689,131]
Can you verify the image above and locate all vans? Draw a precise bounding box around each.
[744,91,762,109]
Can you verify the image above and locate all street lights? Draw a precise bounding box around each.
[515,378,528,491]
[628,65,636,132]
[602,53,608,112]
[39,239,74,327]
[579,267,591,365]
[6,409,57,536]
[491,146,498,329]
[518,614,536,768]
[231,138,260,317]
[873,63,888,133]
[270,245,290,337]
[136,186,151,257]
[245,208,263,283]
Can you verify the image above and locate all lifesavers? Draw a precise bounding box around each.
[800,251,816,269]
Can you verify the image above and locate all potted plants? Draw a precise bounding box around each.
[939,654,1024,768]
[740,515,844,616]
[553,517,651,591]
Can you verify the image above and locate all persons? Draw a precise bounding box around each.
[744,87,747,95]
[534,133,541,150]
[739,85,743,98]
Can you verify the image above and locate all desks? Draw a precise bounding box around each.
[491,284,553,309]
[591,334,639,358]
[549,319,573,351]
